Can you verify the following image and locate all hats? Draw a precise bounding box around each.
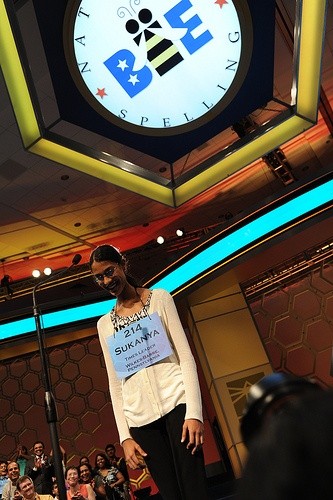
[239,370,319,450]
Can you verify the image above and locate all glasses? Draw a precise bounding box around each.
[93,259,122,282]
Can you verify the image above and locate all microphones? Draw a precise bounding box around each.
[32,253,82,307]
